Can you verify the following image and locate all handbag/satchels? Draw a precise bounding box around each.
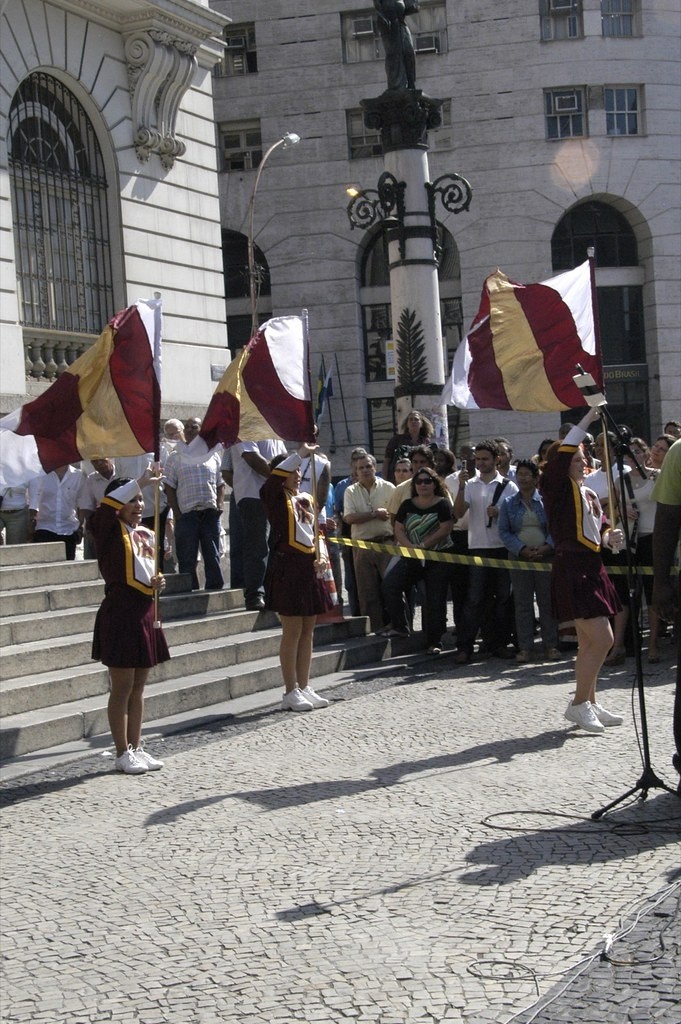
[612,515,639,560]
[381,555,415,594]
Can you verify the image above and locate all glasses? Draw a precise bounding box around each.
[416,478,433,485]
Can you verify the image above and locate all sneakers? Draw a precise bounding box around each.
[375,621,411,638]
[130,740,165,774]
[591,702,623,729]
[281,687,314,712]
[114,744,149,775]
[299,685,329,709]
[564,700,605,733]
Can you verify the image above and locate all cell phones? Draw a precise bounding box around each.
[461,460,467,472]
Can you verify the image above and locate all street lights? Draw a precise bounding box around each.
[251,129,301,334]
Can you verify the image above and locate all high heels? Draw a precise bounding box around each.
[603,643,627,666]
[648,644,660,662]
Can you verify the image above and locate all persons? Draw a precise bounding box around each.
[162,417,224,590]
[0,418,225,573]
[537,405,624,732]
[221,421,681,665]
[381,409,435,480]
[91,460,171,774]
[651,439,680,797]
[259,441,334,711]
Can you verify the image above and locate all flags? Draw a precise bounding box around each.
[0,299,161,488]
[440,256,607,413]
[186,315,314,458]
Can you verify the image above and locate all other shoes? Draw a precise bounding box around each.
[516,649,537,663]
[478,650,495,659]
[425,646,441,655]
[245,595,273,611]
[496,644,513,659]
[544,648,562,660]
[455,651,471,664]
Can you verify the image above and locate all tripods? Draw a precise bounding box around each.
[567,363,681,820]
